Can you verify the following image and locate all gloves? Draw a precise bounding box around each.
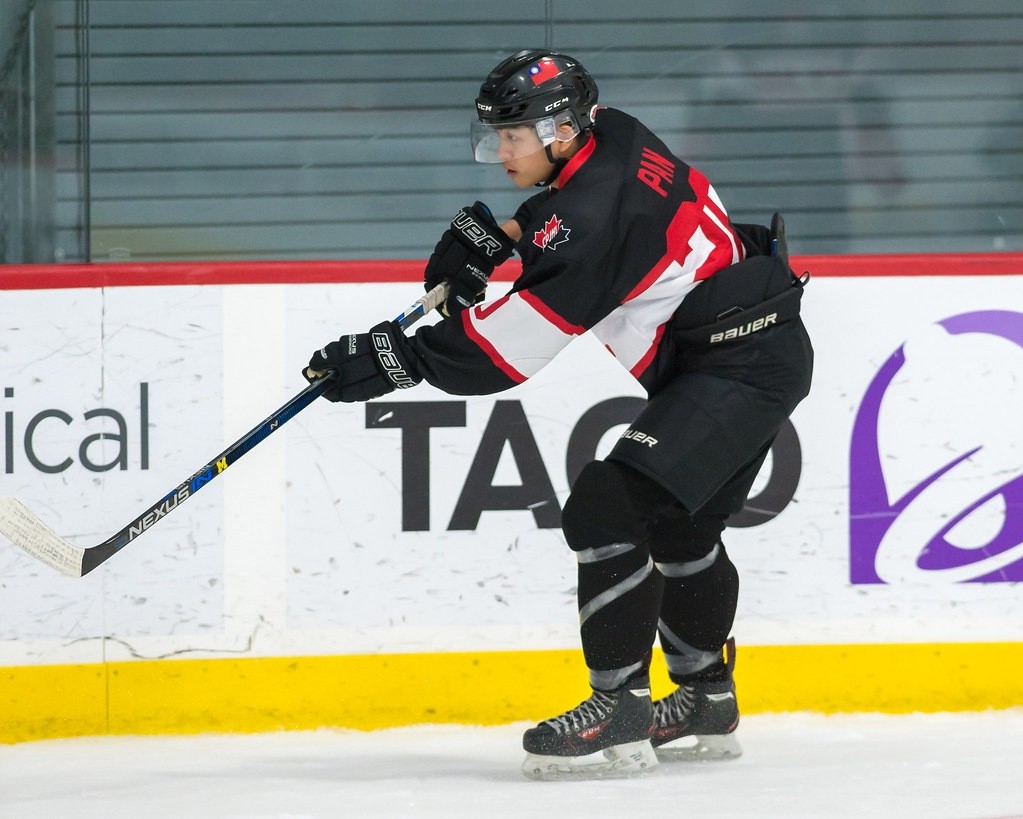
[302,320,434,404]
[425,198,522,318]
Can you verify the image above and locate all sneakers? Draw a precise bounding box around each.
[651,636,745,773]
[520,670,662,776]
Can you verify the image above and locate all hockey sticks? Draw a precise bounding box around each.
[1,276,455,578]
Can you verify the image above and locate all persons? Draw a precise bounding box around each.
[301,48,815,784]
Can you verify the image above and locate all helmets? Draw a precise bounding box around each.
[467,49,600,163]
